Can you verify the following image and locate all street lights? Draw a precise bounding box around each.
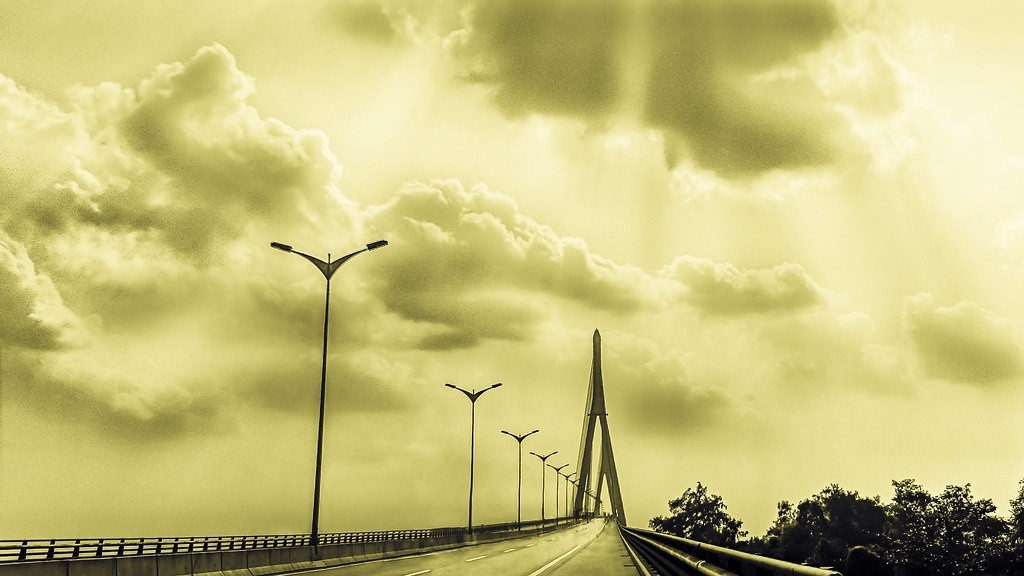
[268,236,389,545]
[546,463,570,525]
[569,478,582,524]
[559,471,577,525]
[529,451,559,528]
[499,429,542,531]
[445,381,503,534]
